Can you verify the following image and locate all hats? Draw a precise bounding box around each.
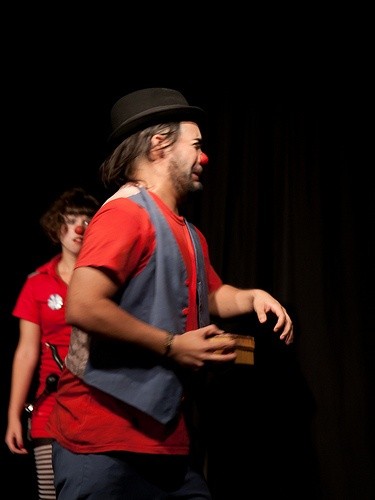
[105,87,205,150]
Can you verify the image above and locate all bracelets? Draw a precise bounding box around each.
[163,332,174,356]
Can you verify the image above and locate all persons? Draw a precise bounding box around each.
[6,188,99,500]
[51,88,294,500]
